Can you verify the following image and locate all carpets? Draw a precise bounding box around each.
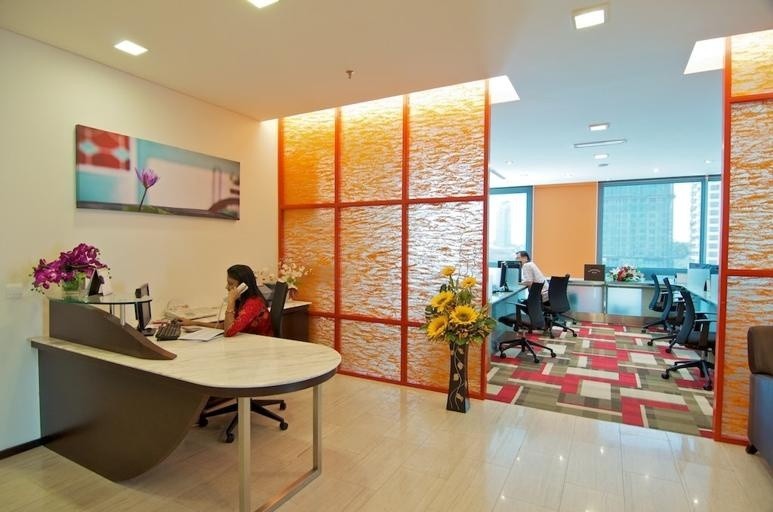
[485,319,717,437]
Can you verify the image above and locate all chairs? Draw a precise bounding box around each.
[526,277,578,339]
[498,280,557,363]
[648,278,685,354]
[662,288,718,391]
[559,274,576,332]
[641,274,686,334]
[197,280,289,442]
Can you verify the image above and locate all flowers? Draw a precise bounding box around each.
[417,266,498,345]
[134,166,158,212]
[28,243,112,298]
[608,262,639,281]
[251,258,315,296]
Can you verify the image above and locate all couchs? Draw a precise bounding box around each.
[745,326,772,467]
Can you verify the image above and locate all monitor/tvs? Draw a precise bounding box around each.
[584,264,605,281]
[499,262,513,292]
[134,282,157,337]
[498,261,521,281]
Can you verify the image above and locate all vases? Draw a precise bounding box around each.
[61,275,93,302]
[443,338,470,413]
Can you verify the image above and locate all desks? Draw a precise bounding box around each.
[545,278,604,323]
[193,298,310,341]
[681,284,719,333]
[605,282,676,326]
[489,284,528,339]
[27,300,343,512]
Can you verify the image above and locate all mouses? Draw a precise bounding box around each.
[170,318,181,323]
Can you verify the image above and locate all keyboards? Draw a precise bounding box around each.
[156,323,181,339]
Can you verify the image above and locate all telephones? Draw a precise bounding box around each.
[223,282,249,302]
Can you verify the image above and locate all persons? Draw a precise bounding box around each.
[514,250,554,331]
[223,264,278,337]
